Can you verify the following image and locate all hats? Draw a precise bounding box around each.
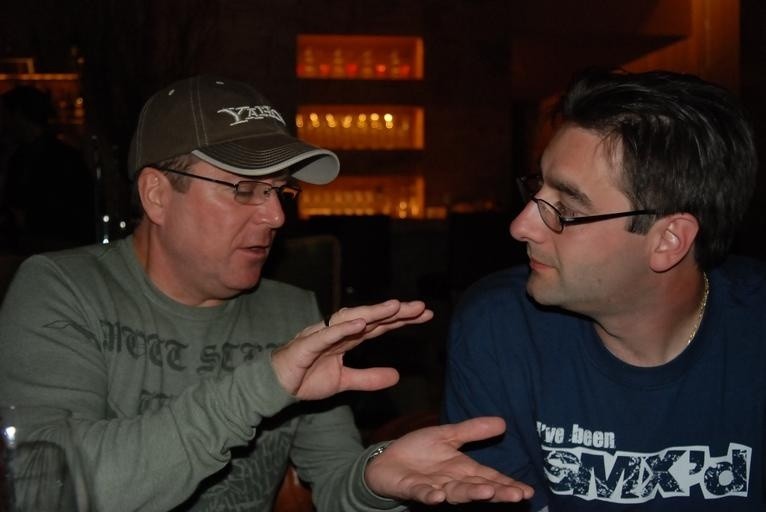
[126,74,341,186]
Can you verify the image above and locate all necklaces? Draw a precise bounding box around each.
[682,272,711,346]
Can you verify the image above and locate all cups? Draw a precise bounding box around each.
[0,402,97,512]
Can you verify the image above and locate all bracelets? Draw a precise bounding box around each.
[368,446,385,461]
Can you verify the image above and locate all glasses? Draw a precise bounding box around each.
[151,166,302,206]
[517,173,658,233]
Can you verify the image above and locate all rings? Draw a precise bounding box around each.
[323,307,346,327]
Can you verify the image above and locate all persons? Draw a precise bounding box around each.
[1,76,534,511]
[440,68,762,509]
